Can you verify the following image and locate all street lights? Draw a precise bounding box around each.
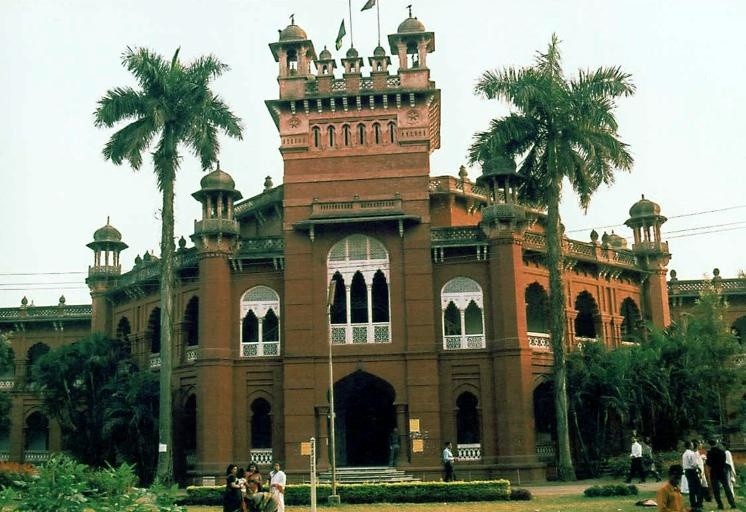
[326,279,340,505]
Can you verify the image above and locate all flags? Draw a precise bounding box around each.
[336,20,346,51]
[361,0,375,12]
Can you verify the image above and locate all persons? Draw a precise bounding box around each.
[682,437,736,512]
[625,436,662,483]
[389,427,401,467]
[223,460,287,512]
[442,441,456,481]
[656,464,692,512]
